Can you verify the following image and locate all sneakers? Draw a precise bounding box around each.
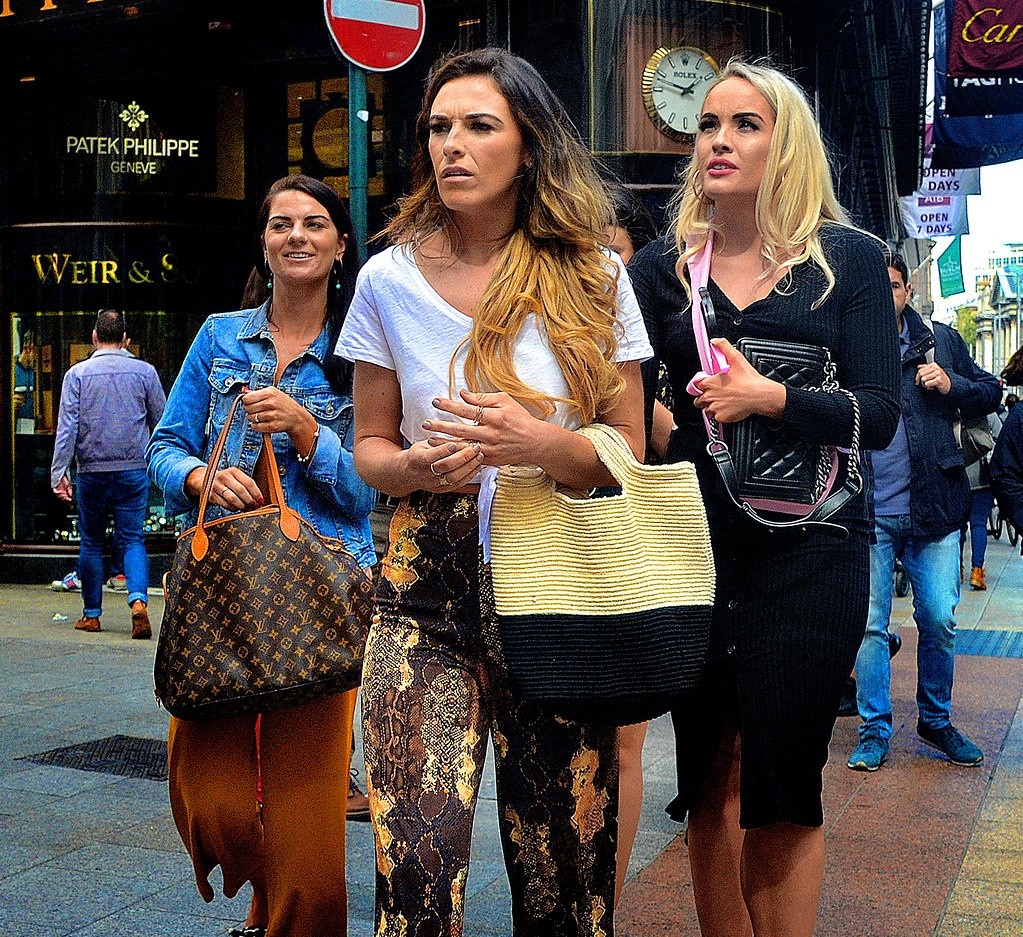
[346,768,370,815]
[917,717,984,767]
[101,577,128,594]
[847,737,890,770]
[967,567,986,590]
[52,571,82,592]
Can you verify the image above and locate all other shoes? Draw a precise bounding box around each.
[131,602,152,639]
[888,632,902,659]
[76,615,100,632]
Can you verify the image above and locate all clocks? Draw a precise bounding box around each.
[645,46,717,144]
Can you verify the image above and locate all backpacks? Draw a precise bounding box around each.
[923,319,993,466]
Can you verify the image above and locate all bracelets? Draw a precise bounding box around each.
[298,423,320,462]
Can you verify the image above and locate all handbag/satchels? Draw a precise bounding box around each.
[728,336,838,516]
[155,394,377,723]
[489,424,715,703]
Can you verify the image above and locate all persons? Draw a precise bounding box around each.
[333,51,656,937]
[349,782,371,817]
[141,179,379,936]
[626,66,901,937]
[50,311,166,638]
[848,253,1004,769]
[14,330,35,419]
[959,392,1023,589]
[605,181,675,899]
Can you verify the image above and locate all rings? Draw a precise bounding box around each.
[474,406,483,423]
[438,475,449,485]
[254,413,259,423]
[220,487,228,496]
[431,464,442,476]
[468,440,480,445]
